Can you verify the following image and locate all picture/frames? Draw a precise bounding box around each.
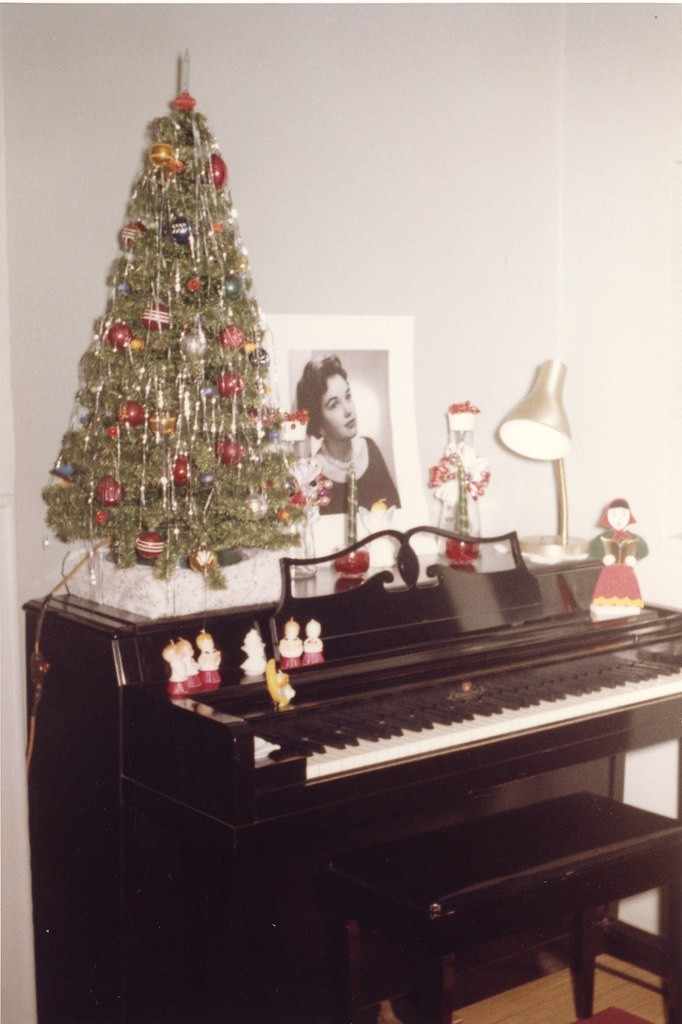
[268,314,438,561]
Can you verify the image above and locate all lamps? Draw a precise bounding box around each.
[499,358,592,559]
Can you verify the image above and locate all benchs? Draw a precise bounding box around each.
[311,790,682,1024]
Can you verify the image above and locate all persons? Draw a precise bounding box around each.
[297,356,402,516]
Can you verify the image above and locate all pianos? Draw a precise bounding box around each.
[22,543,682,1024]
